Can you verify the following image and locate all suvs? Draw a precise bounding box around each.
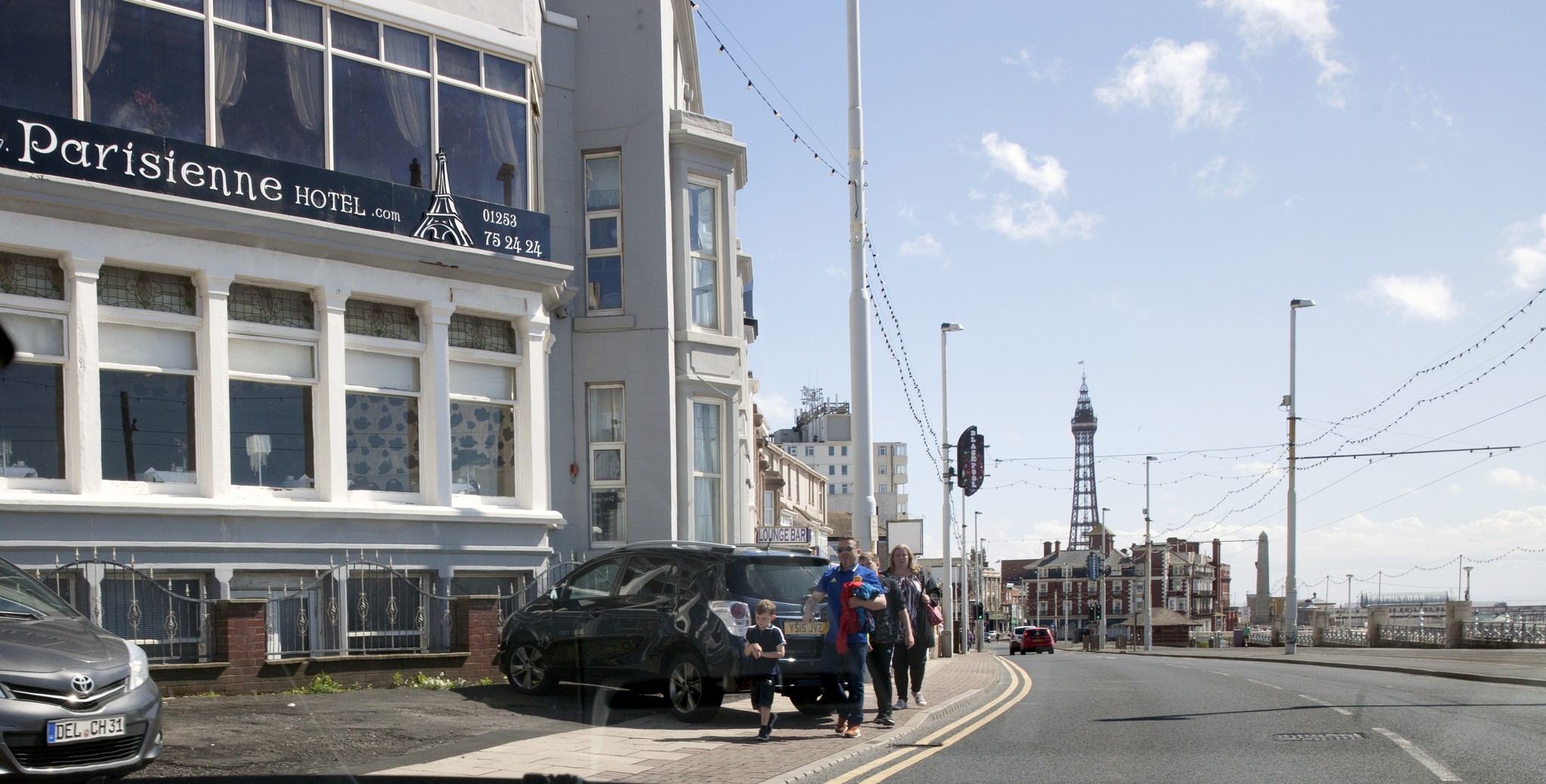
[1008,625,1037,655]
[1018,627,1055,655]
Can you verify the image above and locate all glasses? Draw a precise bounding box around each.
[837,546,858,553]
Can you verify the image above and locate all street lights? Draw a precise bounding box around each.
[1463,565,1474,601]
[1346,574,1354,631]
[1019,577,1024,626]
[1144,456,1158,650]
[1099,508,1111,650]
[1064,562,1068,643]
[1034,571,1040,628]
[975,511,986,652]
[940,322,964,658]
[1285,299,1316,655]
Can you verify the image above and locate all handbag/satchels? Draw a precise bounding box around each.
[919,586,943,627]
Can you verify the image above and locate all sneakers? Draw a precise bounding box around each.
[835,716,848,733]
[911,690,927,706]
[845,724,861,737]
[892,699,907,709]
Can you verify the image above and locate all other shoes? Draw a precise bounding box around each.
[1245,644,1247,648]
[876,712,883,720]
[883,711,895,725]
[768,713,777,735]
[756,729,770,740]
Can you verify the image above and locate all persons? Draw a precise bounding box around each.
[743,599,787,741]
[802,536,887,738]
[996,632,1000,642]
[857,551,915,726]
[1242,624,1252,648]
[879,544,940,709]
[960,628,974,652]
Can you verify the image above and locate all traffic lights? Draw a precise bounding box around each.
[972,605,977,620]
[1088,607,1094,622]
[977,604,983,620]
[1096,605,1101,620]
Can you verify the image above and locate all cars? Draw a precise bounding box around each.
[984,632,991,642]
[988,630,1000,640]
[0,553,163,784]
[491,540,844,724]
